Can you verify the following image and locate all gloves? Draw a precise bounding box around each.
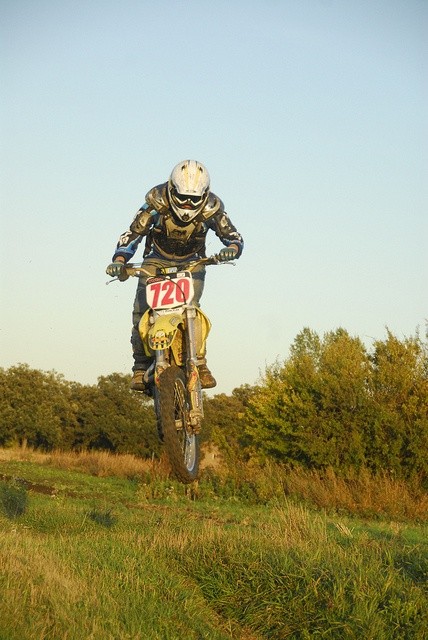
[219,244,238,261]
[106,256,125,277]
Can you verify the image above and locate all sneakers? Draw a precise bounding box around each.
[197,365,217,388]
[131,371,147,390]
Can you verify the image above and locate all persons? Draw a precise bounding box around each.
[110,160,244,392]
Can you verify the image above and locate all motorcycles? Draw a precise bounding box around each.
[106,254,235,483]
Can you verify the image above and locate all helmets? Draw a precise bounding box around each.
[168,160,210,226]
